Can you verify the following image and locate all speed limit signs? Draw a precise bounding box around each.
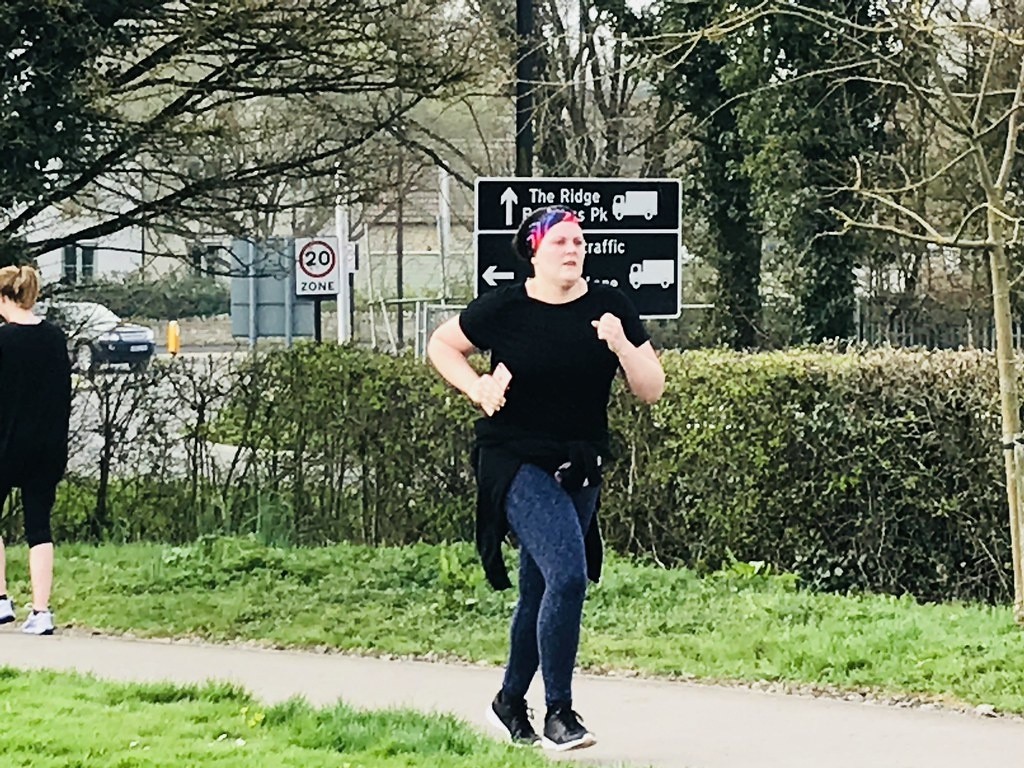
[299,241,336,278]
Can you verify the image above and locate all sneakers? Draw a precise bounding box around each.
[22,612,54,634]
[0,599,15,624]
[542,716,597,752]
[486,691,540,747]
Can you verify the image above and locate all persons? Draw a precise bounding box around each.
[425,206,667,757]
[0,263,74,635]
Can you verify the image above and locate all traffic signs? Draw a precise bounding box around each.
[475,177,681,319]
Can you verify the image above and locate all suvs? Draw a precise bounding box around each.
[32,303,156,374]
